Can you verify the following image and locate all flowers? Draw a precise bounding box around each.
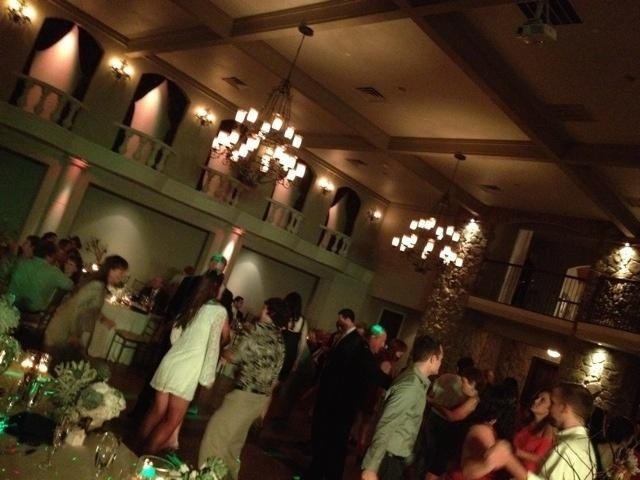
[44,358,127,432]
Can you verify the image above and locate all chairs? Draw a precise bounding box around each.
[25,276,256,372]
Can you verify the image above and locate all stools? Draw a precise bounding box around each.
[384,450,407,463]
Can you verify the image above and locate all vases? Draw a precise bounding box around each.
[44,358,127,432]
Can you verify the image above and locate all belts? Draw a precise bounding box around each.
[91,429,122,480]
[0,339,54,421]
[110,284,152,310]
[36,414,75,473]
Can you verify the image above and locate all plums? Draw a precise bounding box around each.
[512,23,557,45]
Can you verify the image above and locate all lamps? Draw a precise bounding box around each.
[367,207,384,226]
[318,178,336,195]
[210,35,306,186]
[196,105,215,126]
[110,58,131,81]
[25,276,256,372]
[391,157,466,273]
[6,1,37,26]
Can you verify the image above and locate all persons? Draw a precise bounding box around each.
[310,307,640,480]
[128,270,227,455]
[232,295,244,319]
[167,265,195,286]
[141,275,169,309]
[42,255,128,361]
[197,297,291,478]
[1,230,83,313]
[276,291,309,378]
[125,256,234,443]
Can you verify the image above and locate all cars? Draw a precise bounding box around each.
[133,453,176,480]
